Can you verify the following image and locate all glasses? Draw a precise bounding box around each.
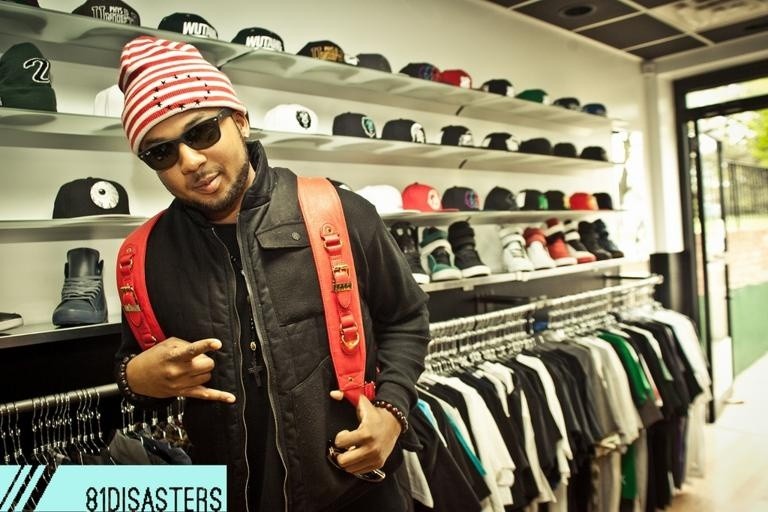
[325,439,386,483]
[137,107,236,171]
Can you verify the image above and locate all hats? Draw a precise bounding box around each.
[296,40,482,91]
[352,182,483,217]
[480,80,606,116]
[52,177,149,219]
[117,35,248,157]
[334,111,394,152]
[70,0,157,40]
[231,28,296,70]
[483,177,613,216]
[1,42,57,112]
[441,125,608,162]
[93,84,125,119]
[382,118,442,155]
[258,104,332,147]
[157,12,236,61]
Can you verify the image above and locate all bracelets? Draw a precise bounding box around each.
[373,397,408,432]
[119,350,144,404]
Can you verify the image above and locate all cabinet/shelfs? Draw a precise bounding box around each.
[0,1,638,349]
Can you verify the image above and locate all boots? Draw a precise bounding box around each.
[389,221,489,284]
[52,247,108,326]
[497,218,624,273]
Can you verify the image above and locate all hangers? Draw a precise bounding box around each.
[0,376,187,464]
[415,274,666,394]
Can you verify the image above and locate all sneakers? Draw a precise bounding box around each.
[0,313,24,332]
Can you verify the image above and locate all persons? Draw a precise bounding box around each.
[110,37,431,512]
